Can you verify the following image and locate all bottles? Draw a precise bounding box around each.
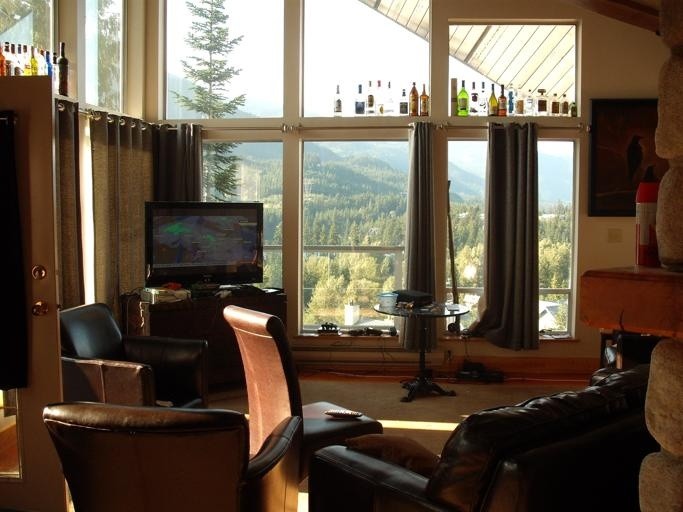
[634,178,661,268]
[354,79,428,117]
[450,77,577,118]
[333,83,342,117]
[0,41,68,97]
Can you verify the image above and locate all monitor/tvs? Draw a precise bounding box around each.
[144,201,264,299]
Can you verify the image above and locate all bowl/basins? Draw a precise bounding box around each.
[377,293,399,307]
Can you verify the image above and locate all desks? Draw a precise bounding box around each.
[372,299,470,405]
[576,266,681,337]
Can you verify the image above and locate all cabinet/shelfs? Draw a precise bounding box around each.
[118,286,289,396]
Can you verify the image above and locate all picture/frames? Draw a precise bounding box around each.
[584,94,664,220]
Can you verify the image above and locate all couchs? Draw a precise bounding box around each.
[307,361,659,510]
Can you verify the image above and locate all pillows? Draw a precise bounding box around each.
[346,434,440,475]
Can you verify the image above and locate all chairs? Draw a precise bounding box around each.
[56,301,212,412]
[222,304,384,512]
[40,400,304,512]
